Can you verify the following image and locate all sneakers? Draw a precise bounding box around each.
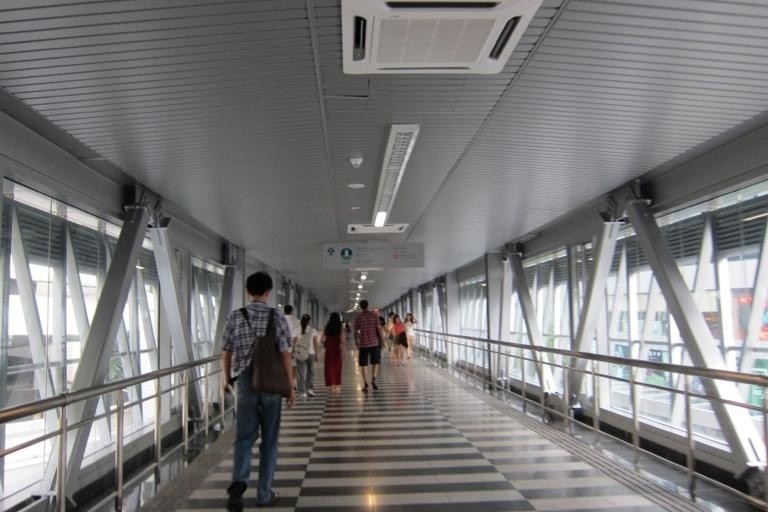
[300,389,315,397]
[363,381,377,391]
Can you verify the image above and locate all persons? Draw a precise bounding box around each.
[283,298,419,397]
[220,272,296,505]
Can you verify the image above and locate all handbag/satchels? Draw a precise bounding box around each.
[251,336,289,397]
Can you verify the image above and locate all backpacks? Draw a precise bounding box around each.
[293,328,312,361]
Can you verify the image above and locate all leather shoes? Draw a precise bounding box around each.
[257,489,279,505]
[227,486,243,512]
